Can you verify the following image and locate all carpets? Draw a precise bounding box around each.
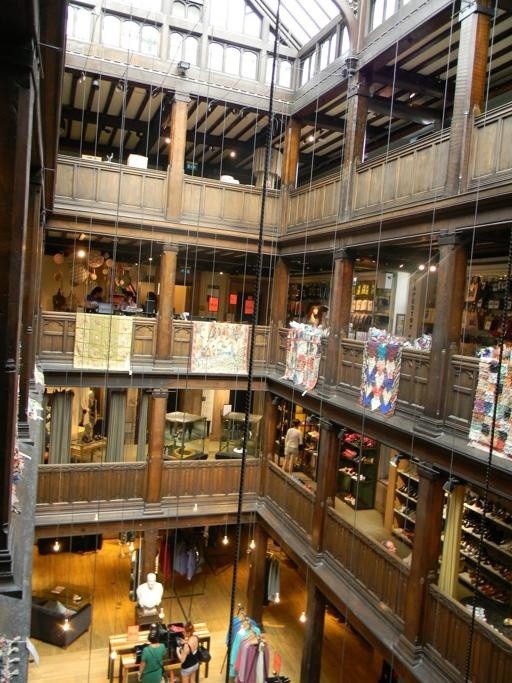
[460,592,512,640]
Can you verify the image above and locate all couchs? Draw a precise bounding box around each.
[30,596,91,649]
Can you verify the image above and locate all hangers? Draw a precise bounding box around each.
[234,603,268,648]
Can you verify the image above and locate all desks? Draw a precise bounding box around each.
[108,623,211,683]
[41,581,95,610]
[70,435,108,463]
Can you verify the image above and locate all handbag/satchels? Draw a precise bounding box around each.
[198,646,211,663]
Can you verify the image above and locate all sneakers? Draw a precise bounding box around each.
[456,484,512,612]
[394,455,420,544]
[299,414,320,454]
[334,426,377,509]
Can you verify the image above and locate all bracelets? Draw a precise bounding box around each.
[138,672,142,676]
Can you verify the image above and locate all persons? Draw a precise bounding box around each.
[136,572,164,609]
[175,624,199,683]
[120,293,137,311]
[381,540,397,553]
[282,419,303,474]
[422,328,433,339]
[313,306,329,330]
[137,631,168,683]
[87,286,104,303]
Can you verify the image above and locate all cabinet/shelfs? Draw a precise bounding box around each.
[276,397,320,483]
[336,428,380,511]
[391,459,511,604]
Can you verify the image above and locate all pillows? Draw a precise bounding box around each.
[55,601,77,619]
